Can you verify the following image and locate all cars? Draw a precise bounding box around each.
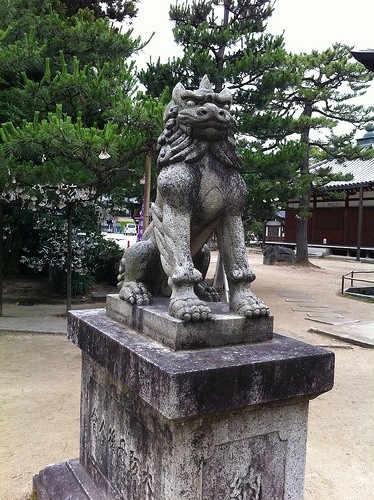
[124,223,136,235]
